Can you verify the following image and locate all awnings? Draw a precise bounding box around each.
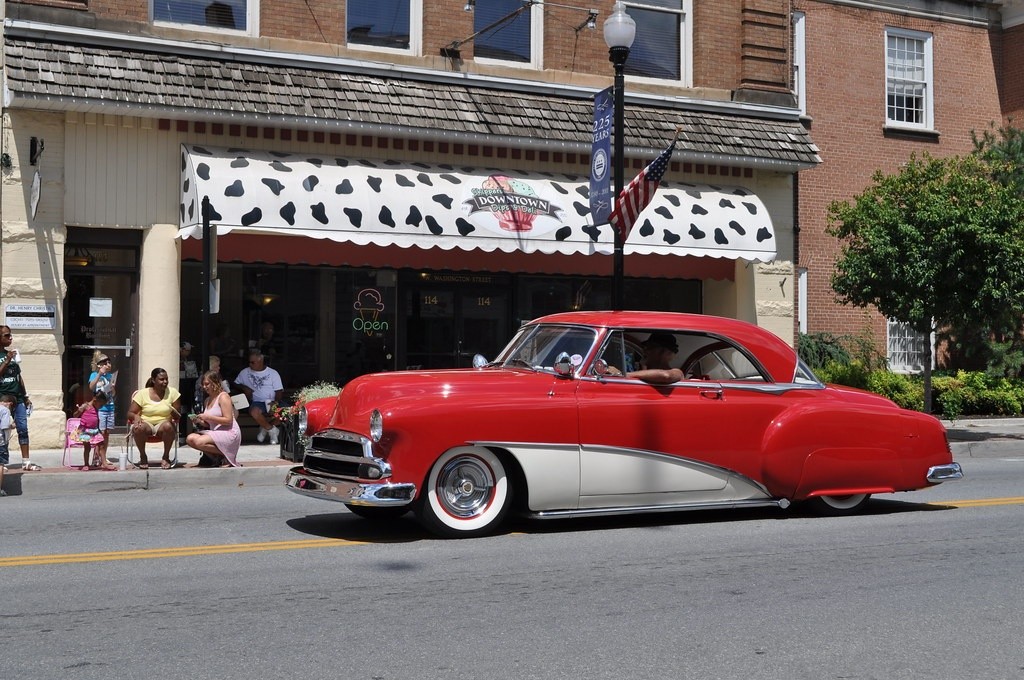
[179,146,777,263]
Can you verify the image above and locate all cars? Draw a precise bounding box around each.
[294,310,961,539]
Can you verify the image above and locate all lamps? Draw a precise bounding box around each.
[64,245,96,273]
[463,0,476,13]
[576,9,599,32]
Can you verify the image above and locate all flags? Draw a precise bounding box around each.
[608,142,676,244]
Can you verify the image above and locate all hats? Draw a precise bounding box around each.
[96,353,110,365]
[637,331,679,355]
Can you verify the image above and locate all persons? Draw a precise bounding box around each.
[0,394,17,496]
[608,332,684,383]
[128,368,181,469]
[180,322,287,468]
[88,354,116,466]
[71,389,119,471]
[0,325,41,470]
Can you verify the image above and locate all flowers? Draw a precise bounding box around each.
[271,381,341,425]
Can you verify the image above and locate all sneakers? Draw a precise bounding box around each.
[267,424,279,445]
[257,426,267,442]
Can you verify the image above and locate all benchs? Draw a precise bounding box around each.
[239,389,297,419]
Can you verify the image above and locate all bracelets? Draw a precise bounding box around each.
[24,393,29,398]
[616,371,622,376]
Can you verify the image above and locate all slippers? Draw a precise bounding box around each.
[139,454,148,469]
[161,456,170,469]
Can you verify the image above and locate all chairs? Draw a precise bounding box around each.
[124,388,180,468]
[62,417,95,469]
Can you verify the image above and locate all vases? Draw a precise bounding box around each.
[280,415,305,462]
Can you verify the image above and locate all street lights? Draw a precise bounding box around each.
[601,0,637,308]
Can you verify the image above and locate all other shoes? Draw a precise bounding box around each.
[218,458,231,468]
[92,458,114,466]
[0,490,7,496]
[82,466,89,471]
[3,466,7,473]
[21,464,42,471]
[101,465,117,470]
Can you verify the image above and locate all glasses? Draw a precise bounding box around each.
[2,333,10,337]
